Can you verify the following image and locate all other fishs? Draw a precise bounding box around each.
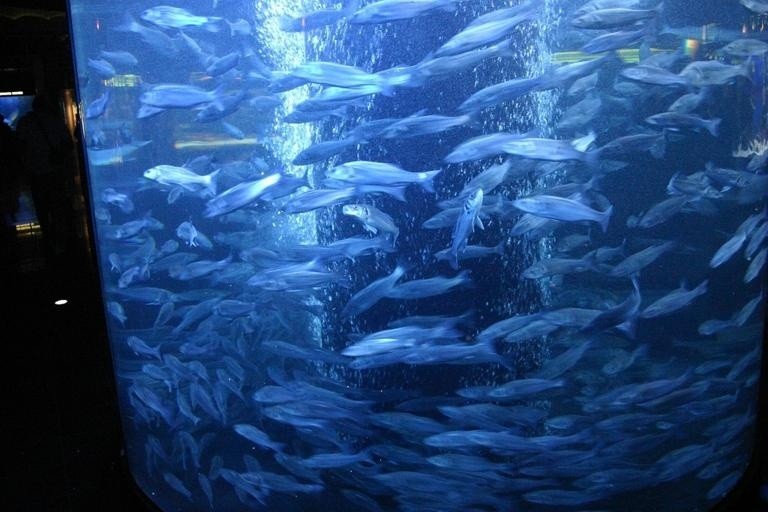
[74,1,767,512]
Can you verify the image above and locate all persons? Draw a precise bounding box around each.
[0,114,23,260]
[15,90,77,266]
